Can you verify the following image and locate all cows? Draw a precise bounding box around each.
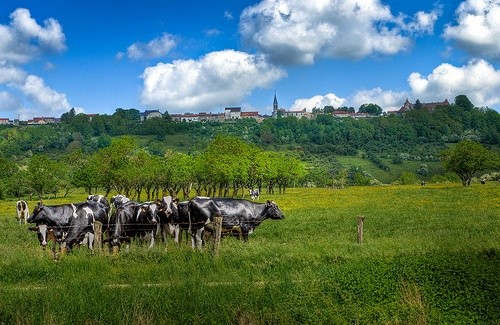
[248,188,261,200]
[15,193,287,264]
[498,179,500,183]
[480,178,486,185]
[420,180,426,186]
